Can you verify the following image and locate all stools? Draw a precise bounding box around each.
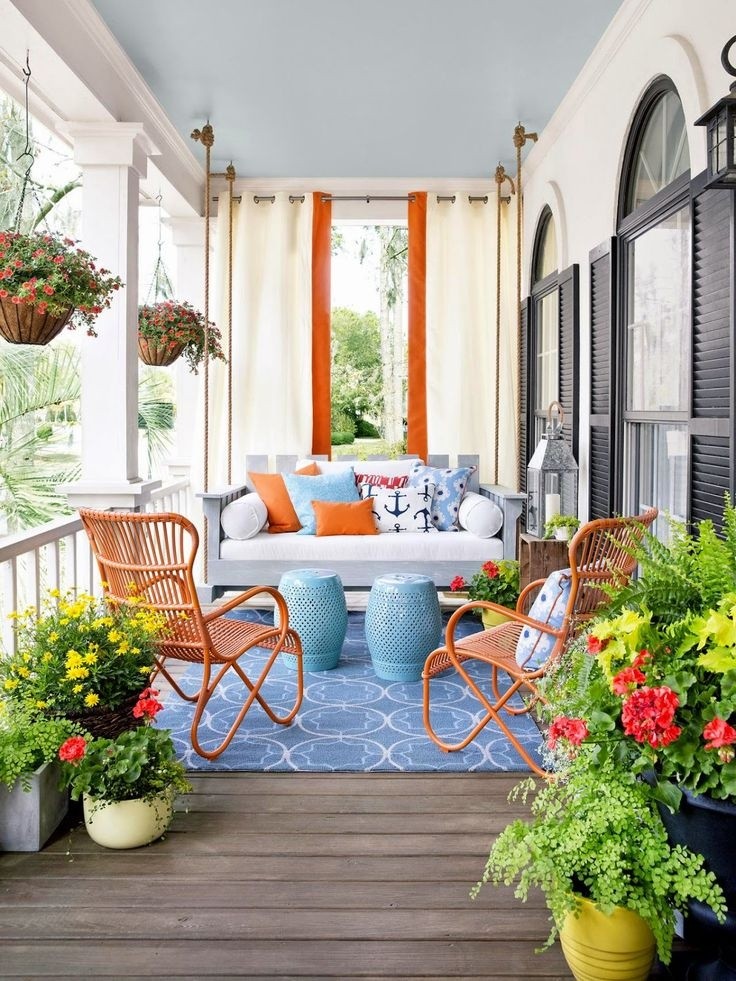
[274,568,348,671]
[364,573,440,682]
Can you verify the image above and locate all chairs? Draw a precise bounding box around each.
[78,506,303,759]
[422,507,658,781]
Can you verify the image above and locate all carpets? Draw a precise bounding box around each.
[144,609,553,774]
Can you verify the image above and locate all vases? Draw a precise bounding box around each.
[548,773,735,981]
[0,289,74,345]
[481,605,516,630]
[18,681,154,747]
[83,784,174,849]
[139,334,185,366]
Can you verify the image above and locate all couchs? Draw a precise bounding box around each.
[194,453,528,609]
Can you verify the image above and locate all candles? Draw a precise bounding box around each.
[545,493,560,535]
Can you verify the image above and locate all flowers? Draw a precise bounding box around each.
[137,300,231,374]
[450,489,736,965]
[0,578,192,707]
[58,687,193,845]
[0,231,125,339]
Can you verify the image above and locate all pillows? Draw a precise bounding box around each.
[250,458,476,536]
[513,557,615,672]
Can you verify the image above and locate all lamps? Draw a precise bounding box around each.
[693,32,736,195]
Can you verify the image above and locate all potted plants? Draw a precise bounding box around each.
[0,700,88,852]
[542,514,582,547]
